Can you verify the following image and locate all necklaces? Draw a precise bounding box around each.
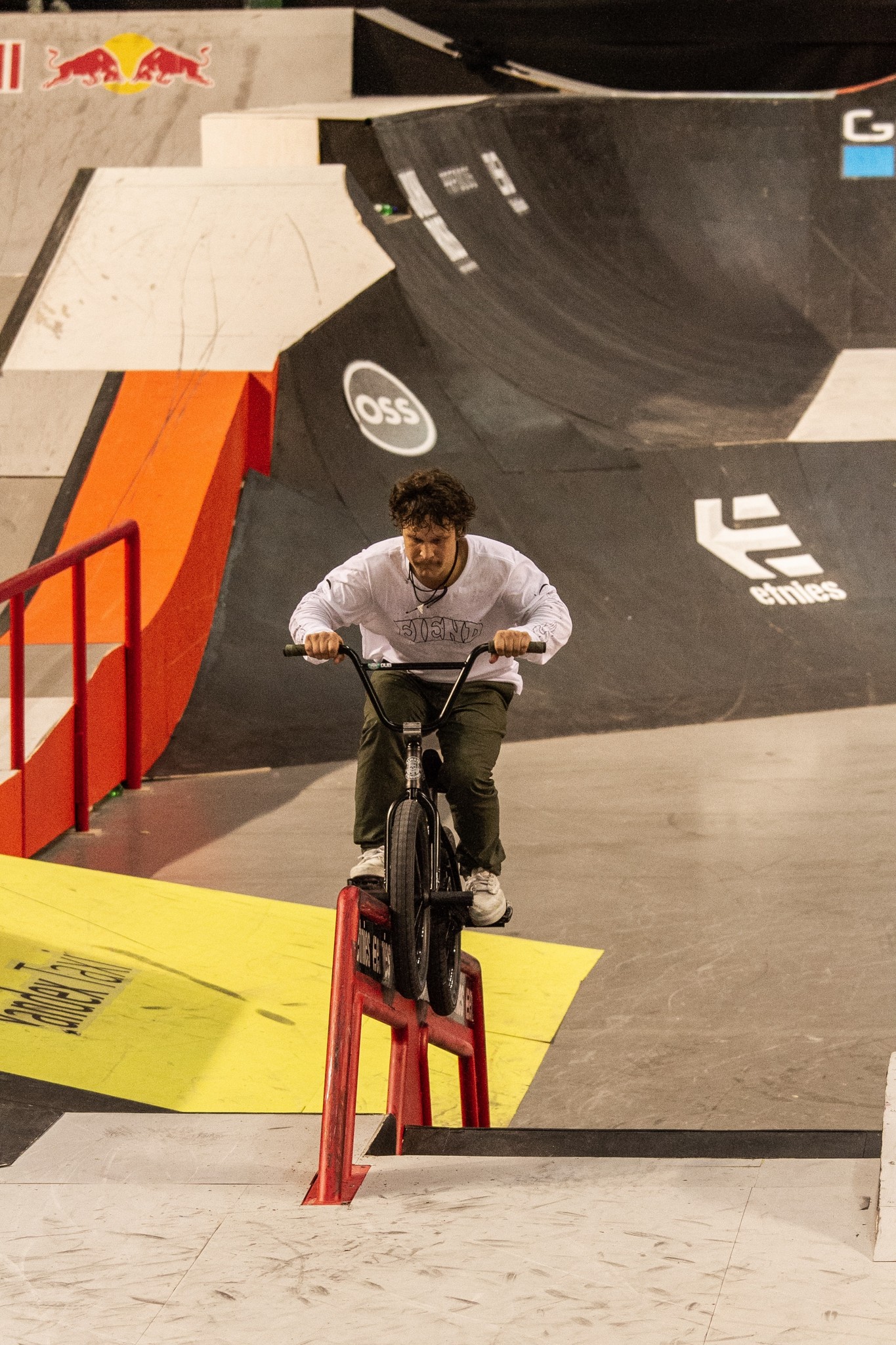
[405,539,459,615]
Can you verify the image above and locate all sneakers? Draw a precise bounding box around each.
[350,845,385,879]
[465,867,506,926]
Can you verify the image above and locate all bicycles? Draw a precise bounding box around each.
[284,642,547,1017]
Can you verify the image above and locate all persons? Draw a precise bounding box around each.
[286,466,573,928]
